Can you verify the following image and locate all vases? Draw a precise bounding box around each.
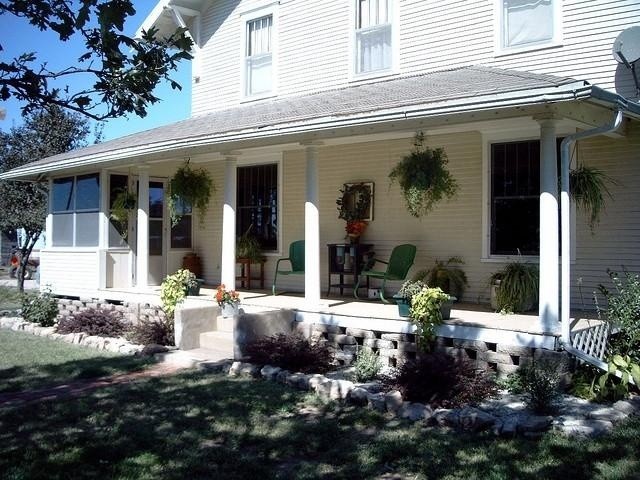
[348,232,361,244]
[220,300,239,317]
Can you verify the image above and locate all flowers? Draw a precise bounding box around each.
[345,220,368,233]
[213,284,240,310]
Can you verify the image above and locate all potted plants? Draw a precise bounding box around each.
[392,255,471,354]
[158,269,201,317]
[489,260,539,314]
[237,233,262,258]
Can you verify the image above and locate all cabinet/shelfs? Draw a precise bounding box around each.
[326,243,374,299]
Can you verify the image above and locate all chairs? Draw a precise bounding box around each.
[354,244,416,304]
[272,239,305,296]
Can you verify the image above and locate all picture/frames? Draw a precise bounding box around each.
[343,182,374,222]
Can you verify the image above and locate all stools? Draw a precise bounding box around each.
[237,258,267,289]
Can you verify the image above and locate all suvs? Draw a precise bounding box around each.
[6,245,40,280]
